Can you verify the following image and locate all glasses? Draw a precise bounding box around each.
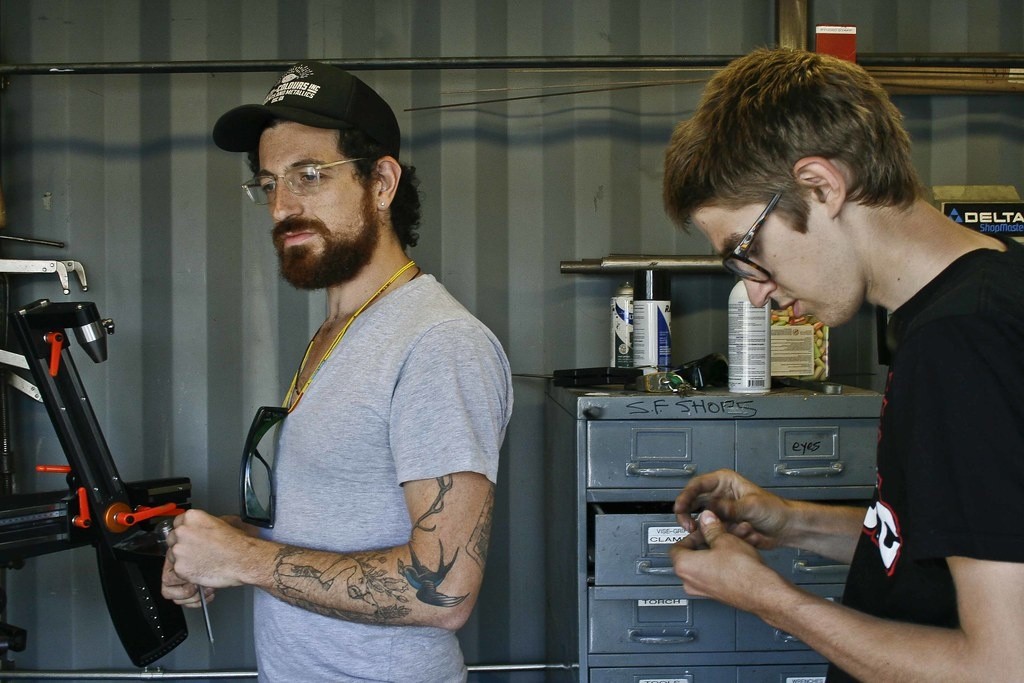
[722,182,787,282]
[240,407,286,528]
[241,155,375,205]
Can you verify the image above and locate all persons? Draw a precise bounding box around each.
[161,62,515,683]
[662,43,1024,683]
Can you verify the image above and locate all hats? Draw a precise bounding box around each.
[213,60,400,161]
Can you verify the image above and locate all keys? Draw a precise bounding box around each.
[661,379,670,385]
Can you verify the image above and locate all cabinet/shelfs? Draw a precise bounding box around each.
[544,379,889,682]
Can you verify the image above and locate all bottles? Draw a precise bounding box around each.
[729,276,771,395]
[770,306,831,387]
[610,280,635,370]
[633,269,671,369]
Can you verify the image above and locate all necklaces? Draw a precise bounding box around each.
[284,260,417,414]
[294,265,423,396]
